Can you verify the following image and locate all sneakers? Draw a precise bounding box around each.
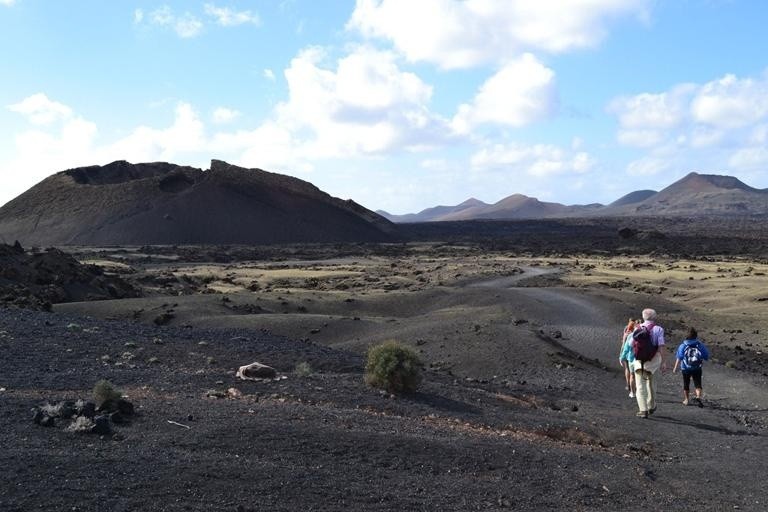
[683,399,689,405]
[695,396,702,406]
[629,392,636,398]
[637,404,656,418]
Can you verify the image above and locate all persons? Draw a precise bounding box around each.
[618,318,644,399]
[672,325,710,411]
[629,308,666,420]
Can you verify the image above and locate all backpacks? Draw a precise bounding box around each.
[684,343,702,369]
[631,325,658,362]
